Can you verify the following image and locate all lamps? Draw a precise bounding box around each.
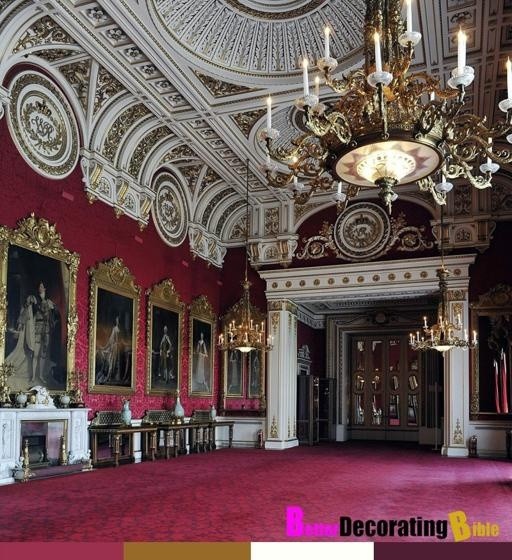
[261,0,512,216]
[218,158,275,354]
[408,205,478,352]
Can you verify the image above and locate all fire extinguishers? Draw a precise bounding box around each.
[256,429,264,449]
[469,433,480,458]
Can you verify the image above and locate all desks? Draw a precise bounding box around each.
[90,421,235,466]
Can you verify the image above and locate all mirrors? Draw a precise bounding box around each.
[469,284,512,421]
[218,298,267,418]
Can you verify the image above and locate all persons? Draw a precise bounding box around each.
[94,314,131,386]
[31,280,61,387]
[156,324,175,384]
[6,293,39,386]
[228,350,241,394]
[193,330,210,386]
[249,349,262,390]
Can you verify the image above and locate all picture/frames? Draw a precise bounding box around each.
[227,350,244,399]
[146,278,186,398]
[87,257,142,395]
[187,294,218,399]
[247,351,261,399]
[0,211,81,397]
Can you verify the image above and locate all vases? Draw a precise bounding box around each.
[174,395,184,417]
[210,404,217,420]
[121,400,131,425]
